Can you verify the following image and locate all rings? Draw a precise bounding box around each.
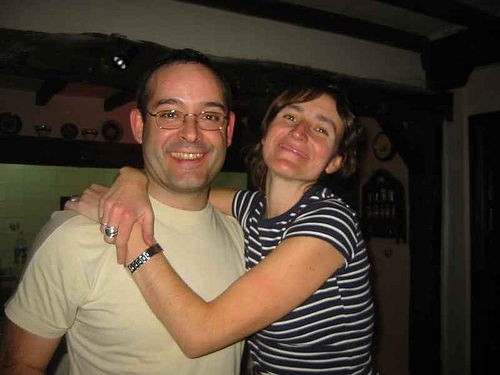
[100,222,118,237]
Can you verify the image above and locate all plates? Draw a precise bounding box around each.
[0,110,24,135]
[57,120,81,140]
[100,119,124,142]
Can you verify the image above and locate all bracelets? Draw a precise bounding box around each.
[128,243,163,273]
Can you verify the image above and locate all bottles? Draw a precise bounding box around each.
[13,230,28,279]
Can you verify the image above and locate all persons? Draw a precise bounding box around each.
[0,48,246,375]
[64,82,379,375]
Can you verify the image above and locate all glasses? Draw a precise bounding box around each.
[145,109,228,130]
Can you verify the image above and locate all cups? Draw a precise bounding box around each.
[34,119,53,137]
[81,128,100,140]
